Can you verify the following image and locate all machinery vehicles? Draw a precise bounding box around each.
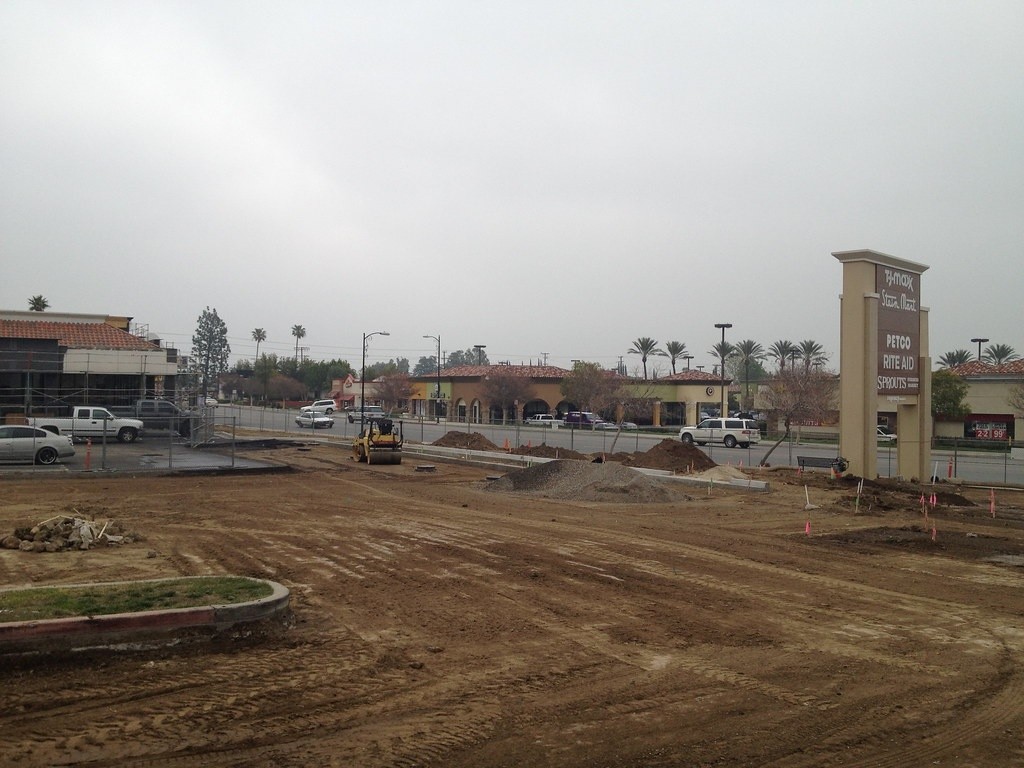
[350,417,403,465]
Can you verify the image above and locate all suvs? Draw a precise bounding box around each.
[24,405,144,444]
[347,404,386,423]
[678,417,762,449]
[524,413,554,425]
[299,399,338,415]
[108,398,203,438]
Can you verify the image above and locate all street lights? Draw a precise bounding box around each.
[684,355,694,370]
[359,331,391,434]
[474,345,487,366]
[970,338,990,361]
[422,334,441,423]
[714,323,733,417]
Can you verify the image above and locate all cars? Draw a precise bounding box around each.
[0,424,76,466]
[294,411,335,429]
[566,410,638,431]
[876,424,898,442]
[206,396,219,408]
